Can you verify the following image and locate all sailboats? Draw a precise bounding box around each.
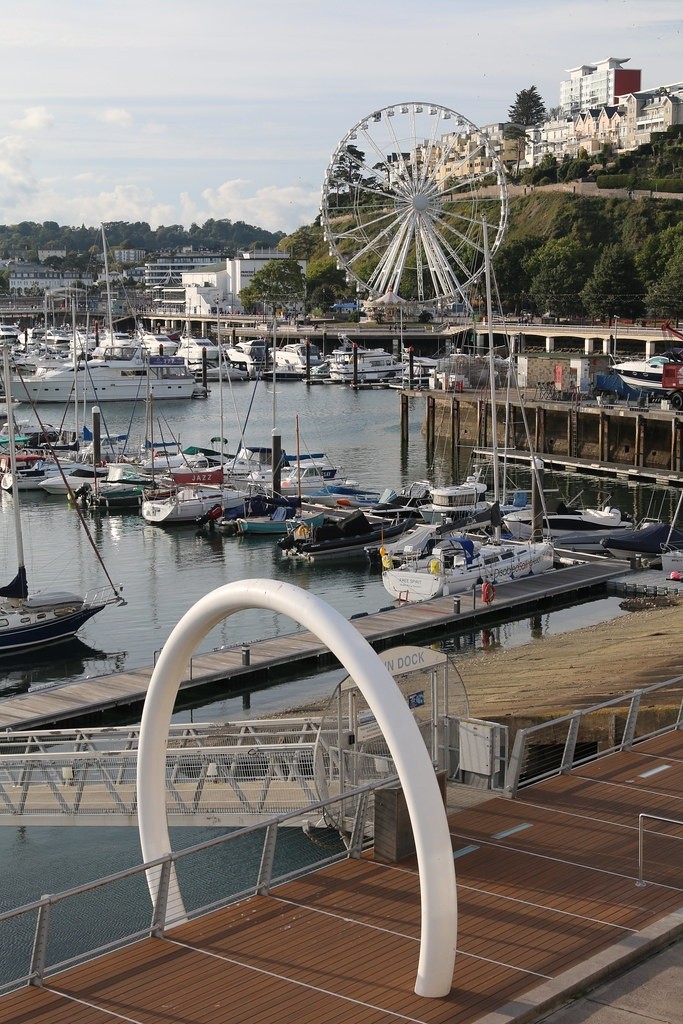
[656,488,683,575]
[10,222,210,403]
[379,212,555,601]
[0,339,127,668]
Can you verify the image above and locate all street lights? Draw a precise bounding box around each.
[521,290,524,324]
[654,291,657,329]
[323,289,325,326]
[229,293,234,315]
[613,315,620,363]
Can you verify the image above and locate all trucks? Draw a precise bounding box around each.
[661,320,683,410]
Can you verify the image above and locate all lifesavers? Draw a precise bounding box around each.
[298,524,309,536]
[482,583,496,602]
[482,632,495,648]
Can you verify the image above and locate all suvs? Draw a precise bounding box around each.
[284,308,300,316]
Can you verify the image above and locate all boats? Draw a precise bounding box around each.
[263,365,306,382]
[309,365,330,378]
[500,488,632,543]
[0,396,157,495]
[66,462,177,507]
[195,502,325,534]
[301,483,380,507]
[225,339,272,363]
[142,437,238,475]
[330,336,407,385]
[247,465,360,497]
[168,447,339,484]
[611,356,677,392]
[600,516,683,561]
[335,480,434,510]
[388,362,463,391]
[322,353,350,363]
[204,359,266,380]
[276,508,416,559]
[273,342,325,368]
[416,468,532,525]
[141,483,251,522]
[0,317,221,362]
